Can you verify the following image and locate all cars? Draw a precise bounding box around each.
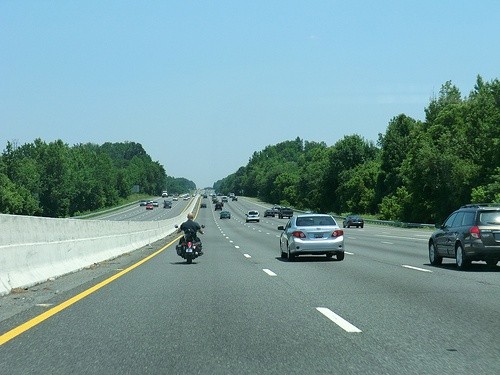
[264,209,274,217]
[220,211,231,219]
[200,192,238,209]
[343,215,364,228]
[272,207,281,213]
[162,190,198,201]
[280,208,294,219]
[215,203,222,210]
[277,213,346,261]
[428,204,500,270]
[139,199,173,210]
[245,210,260,222]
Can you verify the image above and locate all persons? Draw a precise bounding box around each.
[177,212,205,256]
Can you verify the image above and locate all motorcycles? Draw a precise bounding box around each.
[173,224,206,264]
